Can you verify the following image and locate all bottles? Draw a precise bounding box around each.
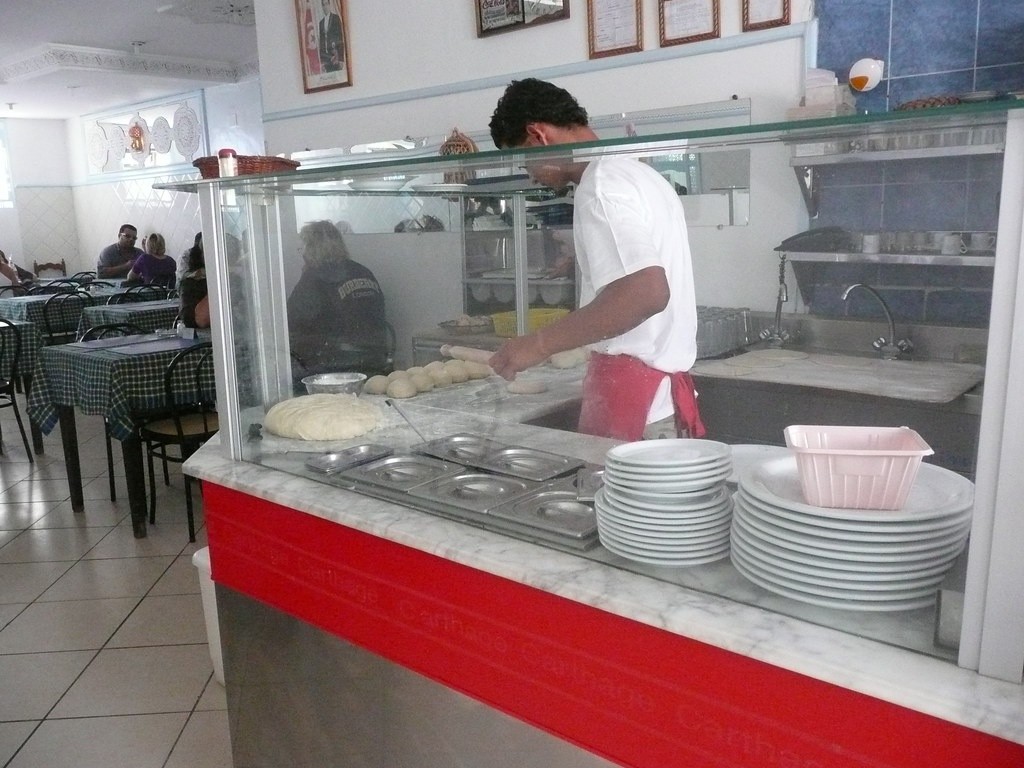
[217,150,239,179]
[176,320,186,338]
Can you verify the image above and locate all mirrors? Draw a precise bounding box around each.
[639,149,750,226]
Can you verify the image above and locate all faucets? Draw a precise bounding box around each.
[759,283,791,348]
[841,283,915,360]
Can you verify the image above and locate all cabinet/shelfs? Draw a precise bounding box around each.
[460,177,582,337]
[780,142,1005,266]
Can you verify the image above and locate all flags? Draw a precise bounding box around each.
[300,0,320,75]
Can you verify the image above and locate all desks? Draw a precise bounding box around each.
[0,318,45,455]
[89,277,129,288]
[75,298,181,344]
[0,285,179,394]
[27,332,303,538]
[19,281,80,294]
[36,276,95,289]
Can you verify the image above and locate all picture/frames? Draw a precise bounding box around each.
[294,0,352,94]
[474,0,571,38]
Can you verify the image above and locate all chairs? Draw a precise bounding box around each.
[0,258,397,544]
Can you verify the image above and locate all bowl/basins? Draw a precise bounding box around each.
[301,373,368,399]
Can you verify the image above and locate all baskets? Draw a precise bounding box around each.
[491,308,570,338]
[439,320,495,336]
[192,155,301,179]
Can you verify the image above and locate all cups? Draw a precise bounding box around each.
[862,231,996,256]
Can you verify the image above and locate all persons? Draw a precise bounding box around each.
[486,78,706,444]
[0,249,38,299]
[174,229,246,329]
[318,0,345,73]
[285,219,390,391]
[126,232,177,289]
[97,223,144,279]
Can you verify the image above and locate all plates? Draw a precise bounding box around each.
[348,179,406,192]
[411,182,470,193]
[593,437,975,613]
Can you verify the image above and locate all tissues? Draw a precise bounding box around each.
[804,67,842,106]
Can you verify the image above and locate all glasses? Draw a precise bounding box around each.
[120,232,137,240]
